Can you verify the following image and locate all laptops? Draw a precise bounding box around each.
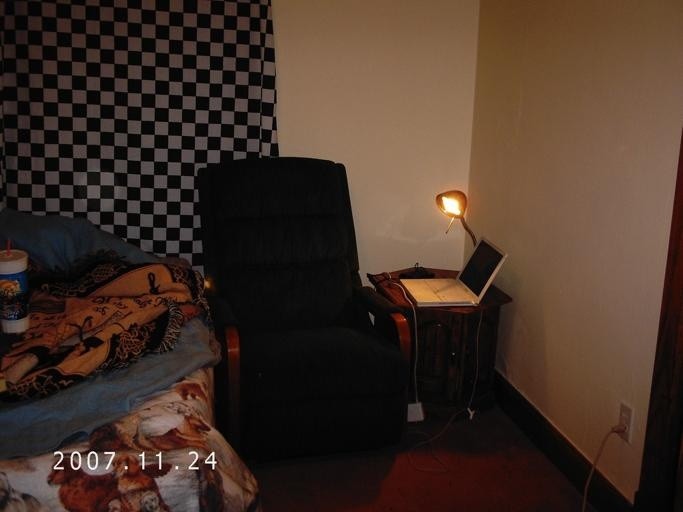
[400,235,508,307]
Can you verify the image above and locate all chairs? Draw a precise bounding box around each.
[196,154,412,462]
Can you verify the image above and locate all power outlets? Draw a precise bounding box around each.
[617,399,635,442]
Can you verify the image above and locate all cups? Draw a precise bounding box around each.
[0,250,30,334]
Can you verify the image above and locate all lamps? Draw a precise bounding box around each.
[436,188,482,259]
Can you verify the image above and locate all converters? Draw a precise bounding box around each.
[406,402,424,421]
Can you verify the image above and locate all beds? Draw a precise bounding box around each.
[2,218,222,511]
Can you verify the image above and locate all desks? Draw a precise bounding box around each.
[365,267,512,418]
